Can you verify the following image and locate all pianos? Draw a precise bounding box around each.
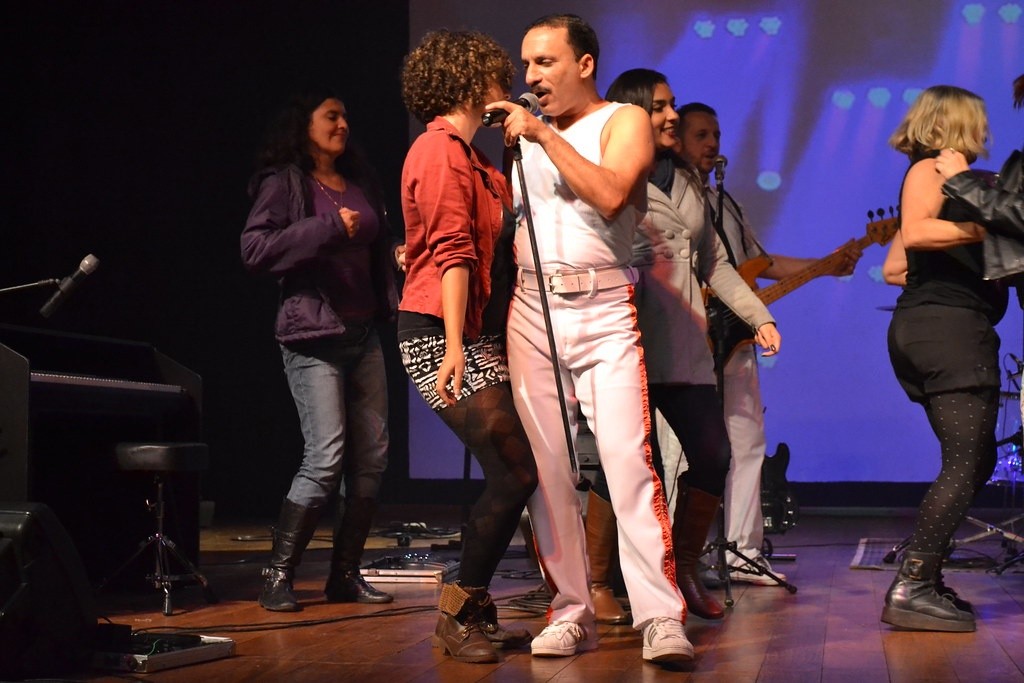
[0,321,209,585]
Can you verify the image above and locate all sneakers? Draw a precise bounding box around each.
[530,618,600,656]
[434,582,533,666]
[643,618,695,665]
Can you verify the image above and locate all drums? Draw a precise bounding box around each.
[1008,419,1023,472]
[986,391,1024,487]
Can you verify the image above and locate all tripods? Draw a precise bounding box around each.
[695,178,798,606]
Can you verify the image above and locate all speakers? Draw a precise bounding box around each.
[0,498,98,683]
[518,418,628,596]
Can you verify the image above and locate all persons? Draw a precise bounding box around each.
[485,14,701,667]
[394,28,532,663]
[881,87,1008,634]
[674,101,788,586]
[235,93,397,612]
[583,68,724,625]
[936,75,1023,309]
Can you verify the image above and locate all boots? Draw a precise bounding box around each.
[880,550,977,631]
[325,494,393,604]
[259,495,329,610]
[585,485,629,624]
[672,476,724,619]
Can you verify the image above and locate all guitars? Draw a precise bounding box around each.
[699,203,901,377]
[761,440,798,534]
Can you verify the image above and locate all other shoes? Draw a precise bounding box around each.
[729,559,787,587]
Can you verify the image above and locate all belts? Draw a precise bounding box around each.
[521,264,639,294]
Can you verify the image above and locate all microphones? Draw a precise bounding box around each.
[712,155,729,180]
[1009,353,1024,371]
[41,254,99,317]
[481,93,540,127]
